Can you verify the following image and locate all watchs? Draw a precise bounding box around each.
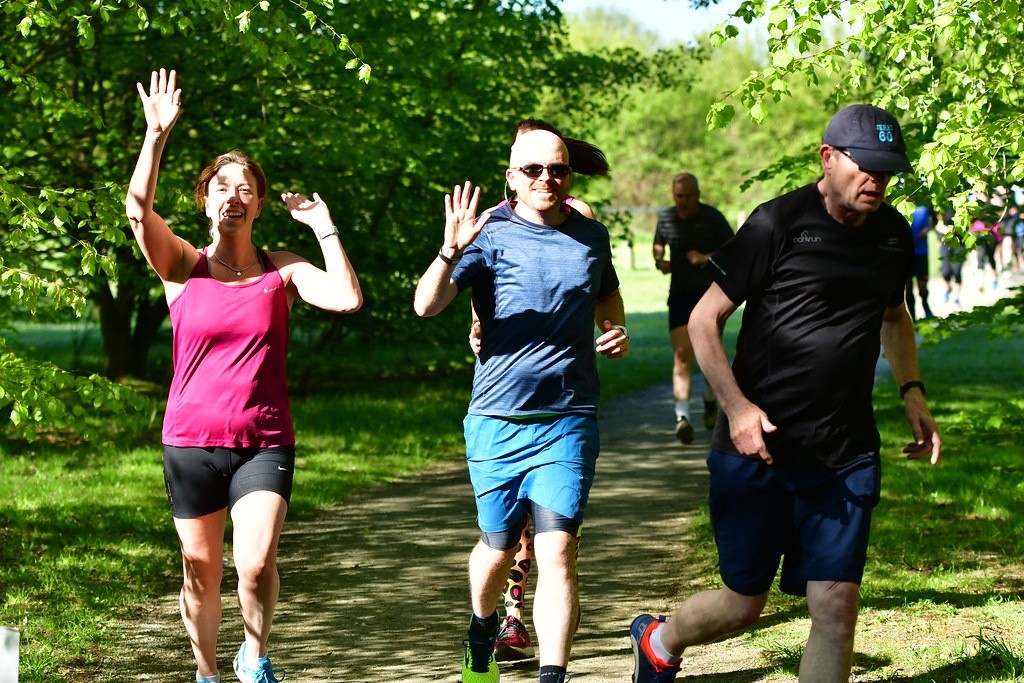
[613,324,629,342]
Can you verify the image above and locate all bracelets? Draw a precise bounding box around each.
[438,246,464,265]
[317,226,338,240]
[472,319,480,327]
[899,380,925,401]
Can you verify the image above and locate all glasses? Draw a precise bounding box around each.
[509,163,570,178]
[833,147,897,176]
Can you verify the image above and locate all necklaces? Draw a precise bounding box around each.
[211,242,258,276]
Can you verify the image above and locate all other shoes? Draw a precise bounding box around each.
[703,401,717,430]
[991,282,997,292]
[943,287,952,303]
[675,416,694,445]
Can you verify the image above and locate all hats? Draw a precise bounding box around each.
[823,103,915,175]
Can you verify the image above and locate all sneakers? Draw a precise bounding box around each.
[462,609,500,683]
[631,614,683,683]
[233,641,286,683]
[493,615,535,662]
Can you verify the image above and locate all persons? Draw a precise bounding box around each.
[653,172,735,445]
[414,129,628,683]
[126,67,363,683]
[470,118,610,667]
[900,168,1024,323]
[628,104,941,683]
